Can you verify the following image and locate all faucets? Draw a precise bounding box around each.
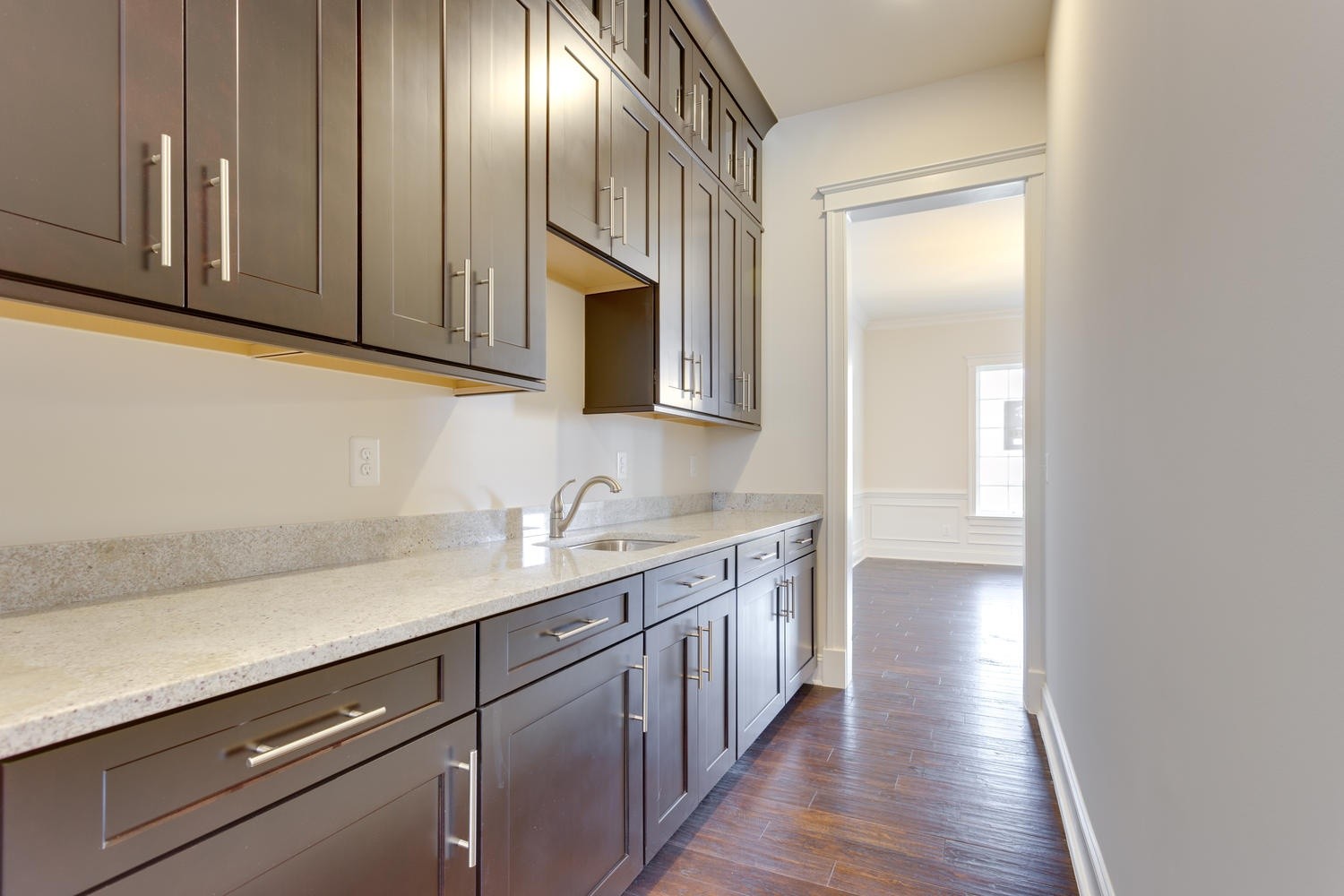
[549,475,623,536]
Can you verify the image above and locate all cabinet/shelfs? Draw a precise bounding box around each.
[0,521,818,896]
[0,0,778,435]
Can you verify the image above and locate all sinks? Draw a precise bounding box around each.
[532,531,701,551]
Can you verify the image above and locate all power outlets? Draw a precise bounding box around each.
[616,451,627,480]
[689,456,698,476]
[349,440,379,488]
